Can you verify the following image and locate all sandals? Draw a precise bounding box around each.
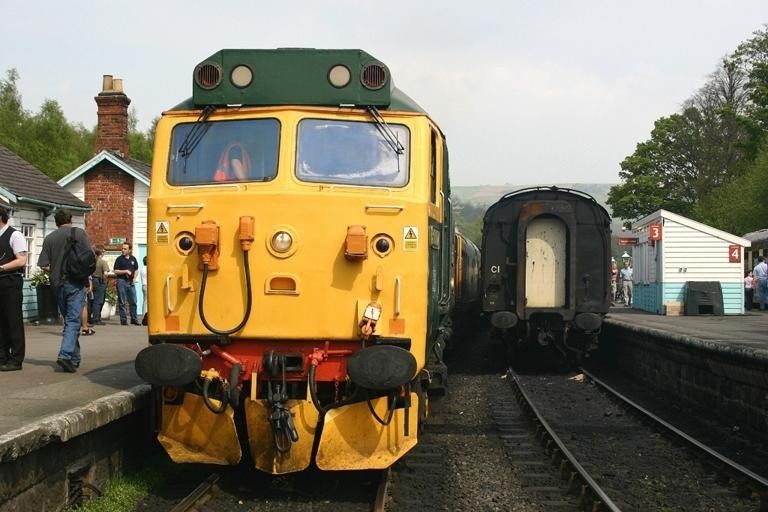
[82,329,95,335]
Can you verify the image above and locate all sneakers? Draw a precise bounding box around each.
[0,361,22,371]
[131,319,141,325]
[56,359,79,372]
[121,320,128,325]
[87,320,105,326]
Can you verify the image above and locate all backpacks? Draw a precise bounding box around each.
[60,227,97,281]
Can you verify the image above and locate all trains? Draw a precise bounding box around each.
[132,45,479,498]
[476,185,615,375]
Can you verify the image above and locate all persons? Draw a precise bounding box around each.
[752,256,768,310]
[86,244,110,325]
[744,270,754,310]
[37,208,97,372]
[0,205,28,371]
[140,256,149,319]
[81,276,95,336]
[114,241,142,325]
[620,261,633,307]
[212,127,260,182]
[612,263,618,306]
